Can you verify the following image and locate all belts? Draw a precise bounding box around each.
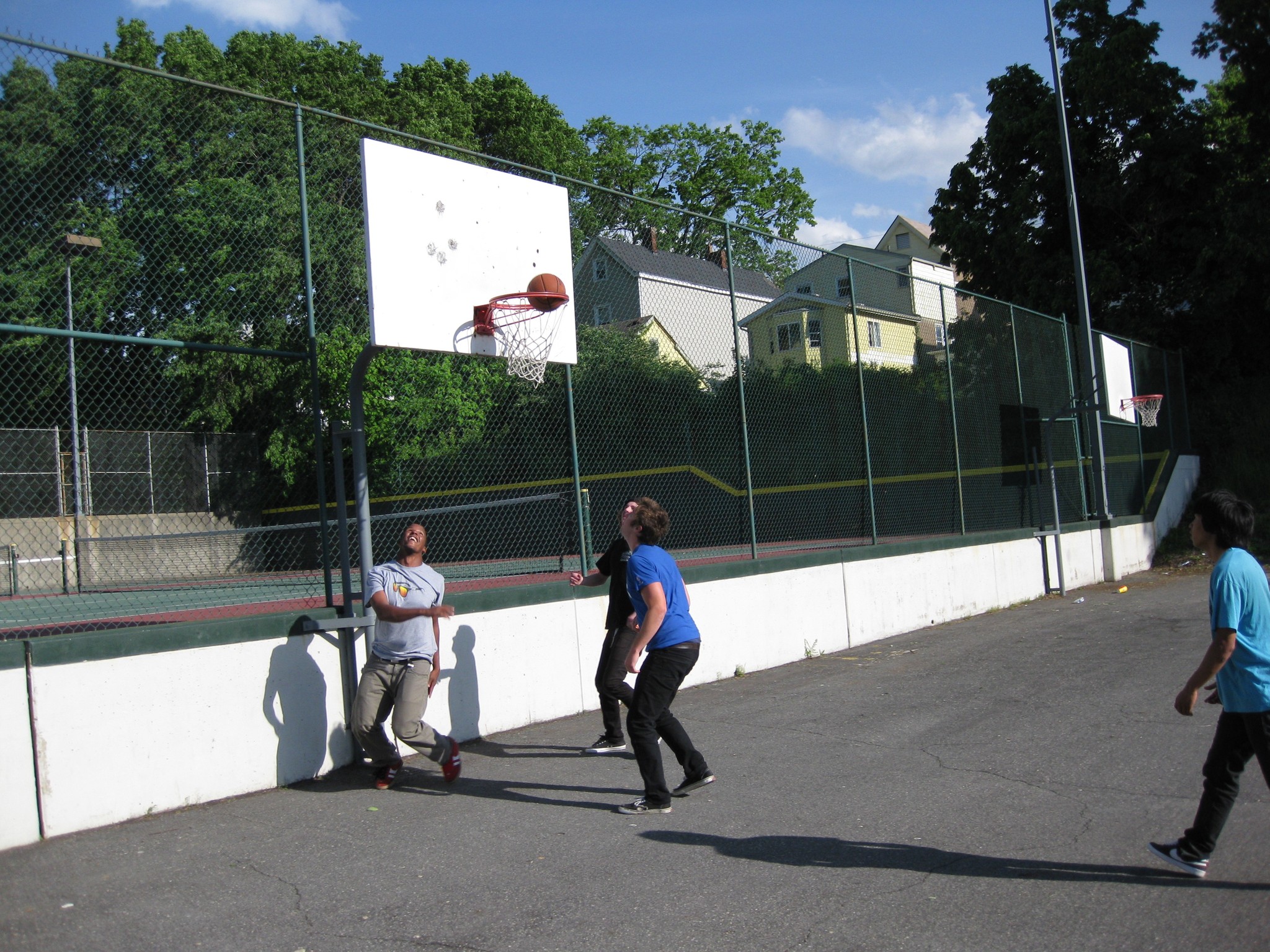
[381,657,428,667]
[670,643,699,650]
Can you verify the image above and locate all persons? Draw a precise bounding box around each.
[569,499,662,755]
[1149,490,1269,877]
[617,497,716,814]
[349,523,461,791]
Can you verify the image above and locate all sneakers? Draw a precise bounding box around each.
[618,797,672,815]
[672,769,715,796]
[373,758,403,789]
[442,735,460,784]
[653,729,662,744]
[585,734,626,753]
[1148,841,1208,877]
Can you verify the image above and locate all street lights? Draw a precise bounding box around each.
[48,233,103,596]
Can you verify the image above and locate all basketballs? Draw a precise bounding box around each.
[527,273,566,312]
